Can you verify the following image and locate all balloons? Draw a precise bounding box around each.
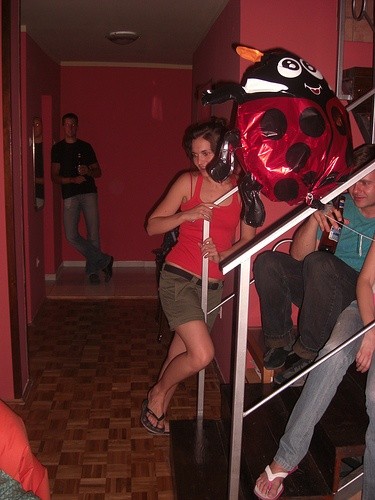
[202,46,373,227]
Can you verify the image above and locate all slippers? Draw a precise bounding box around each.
[140,399,165,433]
[254,466,298,500]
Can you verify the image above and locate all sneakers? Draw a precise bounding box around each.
[274,358,314,386]
[264,347,289,369]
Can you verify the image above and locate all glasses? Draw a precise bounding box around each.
[64,123,76,128]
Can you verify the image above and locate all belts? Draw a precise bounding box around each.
[164,264,224,290]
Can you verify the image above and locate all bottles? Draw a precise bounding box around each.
[75,153,81,177]
[318,196,346,254]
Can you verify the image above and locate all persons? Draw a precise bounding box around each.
[34,119,44,209]
[256,242,375,500]
[142,118,256,436]
[52,112,114,284]
[254,143,375,386]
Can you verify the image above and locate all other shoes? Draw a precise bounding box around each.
[90,274,99,284]
[103,256,114,282]
